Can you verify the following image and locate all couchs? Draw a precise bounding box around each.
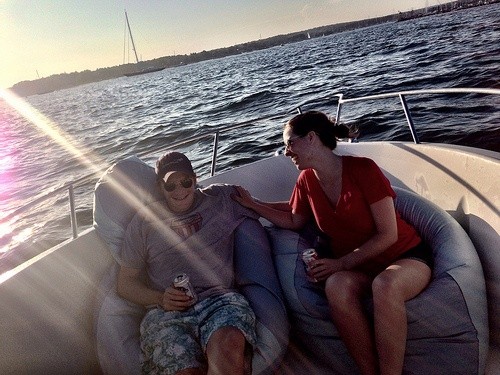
[274,185,489,375]
[97,218,288,375]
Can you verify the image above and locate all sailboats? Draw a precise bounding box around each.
[119,10,166,77]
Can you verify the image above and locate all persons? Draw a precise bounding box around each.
[232,109,435,375]
[116,151,291,375]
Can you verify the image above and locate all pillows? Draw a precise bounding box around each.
[92,157,164,267]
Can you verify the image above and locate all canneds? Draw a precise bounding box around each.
[299,248,320,283]
[172,273,198,308]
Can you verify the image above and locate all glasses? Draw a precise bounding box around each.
[284,134,300,147]
[161,175,192,192]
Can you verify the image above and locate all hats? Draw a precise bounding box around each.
[155,152,192,179]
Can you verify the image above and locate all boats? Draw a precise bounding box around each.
[0,87,500,375]
